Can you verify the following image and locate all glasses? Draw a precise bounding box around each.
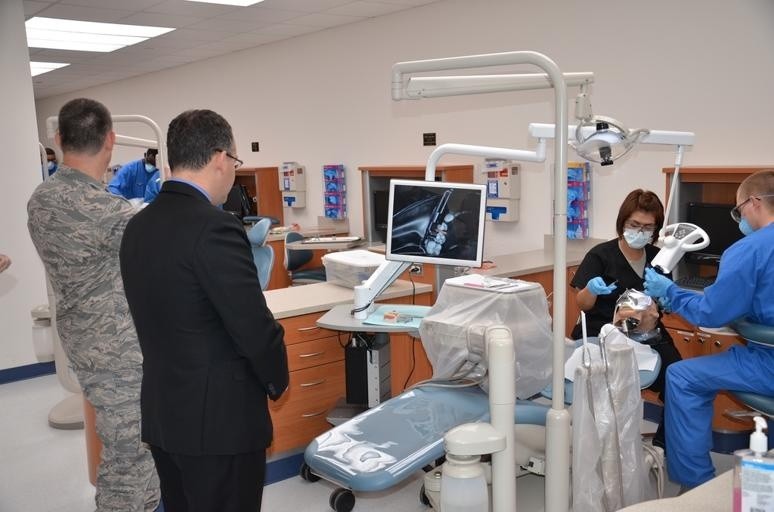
[215,149,243,171]
[730,198,760,223]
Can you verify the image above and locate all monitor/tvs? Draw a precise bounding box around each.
[385,178,487,268]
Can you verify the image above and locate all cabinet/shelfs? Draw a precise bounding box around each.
[639,167,774,432]
[252,279,435,465]
[466,243,597,340]
[227,165,290,288]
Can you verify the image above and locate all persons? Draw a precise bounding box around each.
[40,148,58,178]
[25,97,162,511]
[143,163,161,205]
[104,146,156,200]
[612,290,659,338]
[118,109,291,510]
[644,166,772,499]
[569,189,683,456]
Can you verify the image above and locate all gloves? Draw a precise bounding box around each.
[587,277,617,296]
[642,267,673,297]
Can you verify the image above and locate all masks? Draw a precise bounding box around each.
[623,228,654,249]
[48,161,55,170]
[739,195,761,235]
[145,163,156,173]
